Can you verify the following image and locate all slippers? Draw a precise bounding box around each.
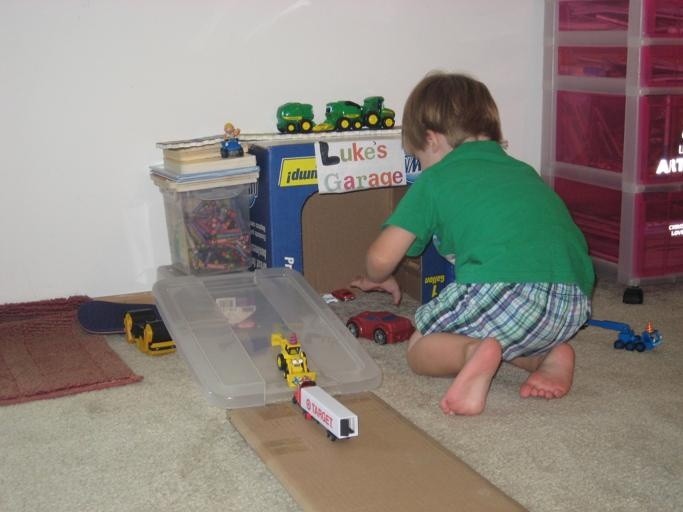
[77,300,159,335]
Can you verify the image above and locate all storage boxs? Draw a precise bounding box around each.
[159,183,252,277]
[248,131,457,304]
[157,265,273,354]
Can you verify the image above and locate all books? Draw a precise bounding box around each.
[149,123,261,192]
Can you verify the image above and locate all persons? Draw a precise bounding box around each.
[350,71,596,417]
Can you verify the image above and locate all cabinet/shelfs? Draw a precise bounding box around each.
[540,0,683,304]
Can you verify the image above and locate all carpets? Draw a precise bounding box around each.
[0,295,143,406]
